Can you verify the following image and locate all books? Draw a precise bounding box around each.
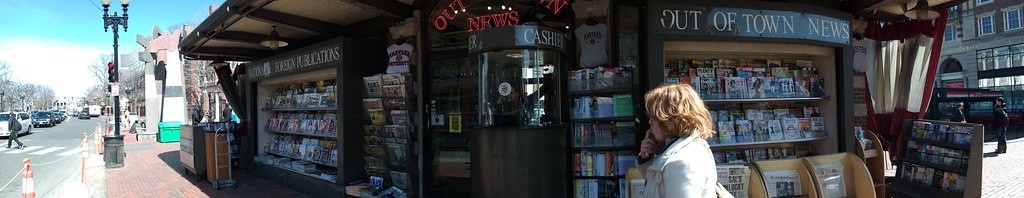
[663,57,825,164]
[345,73,415,198]
[900,121,975,195]
[259,85,337,175]
[568,66,645,198]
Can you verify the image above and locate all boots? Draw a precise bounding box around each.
[1001,144,1008,153]
[994,145,1002,154]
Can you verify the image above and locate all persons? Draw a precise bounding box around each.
[223,103,232,122]
[992,97,1009,154]
[124,108,130,119]
[950,102,966,123]
[634,81,734,198]
[6,112,24,150]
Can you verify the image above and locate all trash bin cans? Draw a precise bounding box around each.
[158,121,183,143]
[102,134,126,167]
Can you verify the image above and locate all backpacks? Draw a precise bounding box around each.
[9,116,23,131]
[997,108,1010,127]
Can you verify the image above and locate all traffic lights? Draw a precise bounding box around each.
[106,62,114,82]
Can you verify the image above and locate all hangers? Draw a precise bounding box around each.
[584,10,598,26]
[394,34,404,46]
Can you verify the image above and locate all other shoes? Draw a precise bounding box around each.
[20,143,24,150]
[6,146,12,149]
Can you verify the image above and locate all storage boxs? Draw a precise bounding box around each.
[439,151,470,178]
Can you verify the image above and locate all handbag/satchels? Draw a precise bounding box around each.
[716,181,735,198]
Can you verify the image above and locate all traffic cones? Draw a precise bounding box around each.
[21,159,36,198]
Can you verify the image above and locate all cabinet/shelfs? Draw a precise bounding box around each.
[895,118,984,198]
[565,66,643,198]
[854,129,886,198]
[662,39,837,155]
[192,121,240,168]
[256,66,338,184]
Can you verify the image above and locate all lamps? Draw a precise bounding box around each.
[260,25,289,49]
[903,0,939,20]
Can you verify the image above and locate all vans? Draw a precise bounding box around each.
[0,111,34,137]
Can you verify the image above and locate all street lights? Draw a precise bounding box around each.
[99,0,131,133]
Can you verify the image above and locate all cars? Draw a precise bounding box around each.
[31,110,57,128]
[53,112,62,124]
[53,108,68,121]
[78,111,90,119]
[72,111,80,117]
